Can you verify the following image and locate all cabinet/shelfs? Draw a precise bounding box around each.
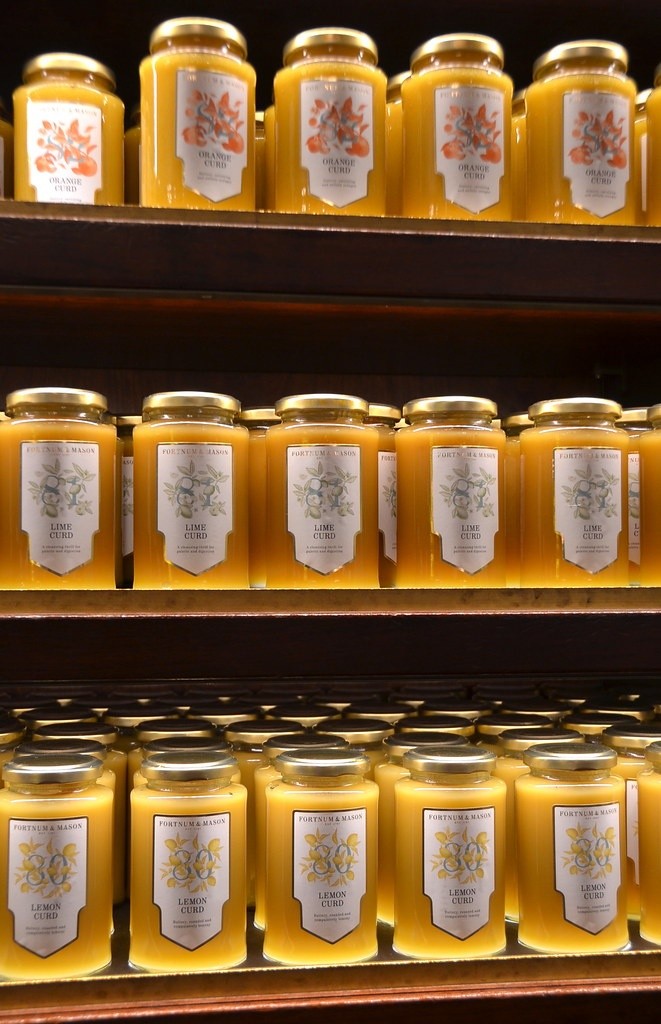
[0,198,661,1024]
